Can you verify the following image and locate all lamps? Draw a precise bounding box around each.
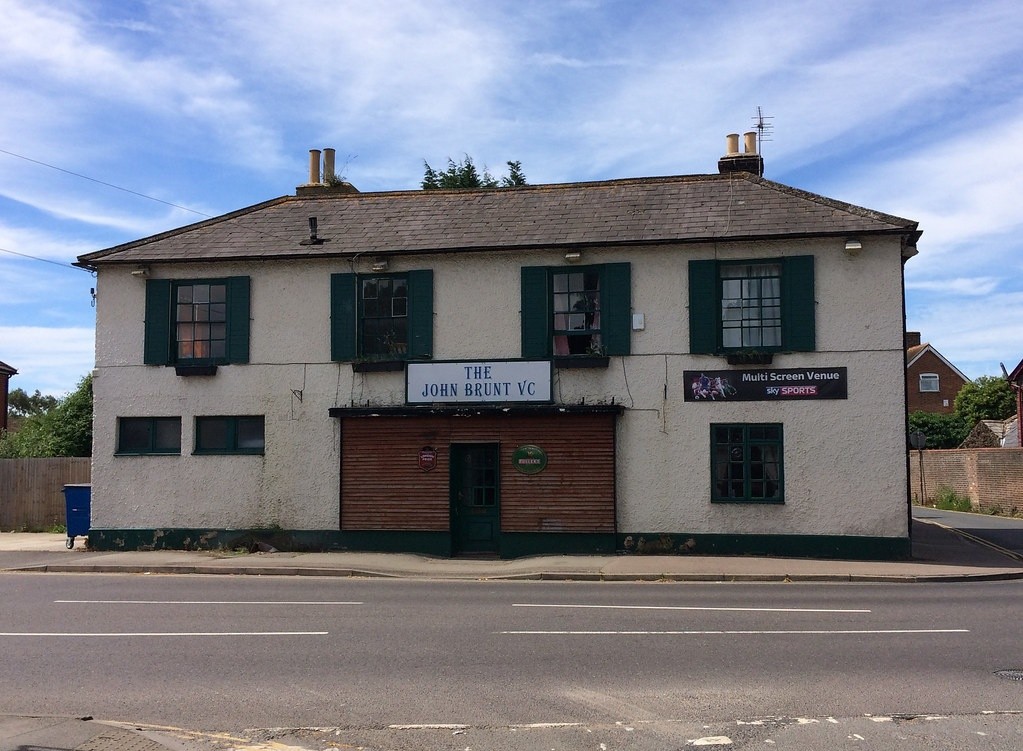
[844,240,862,249]
[371,258,389,271]
[565,249,582,263]
[131,268,150,278]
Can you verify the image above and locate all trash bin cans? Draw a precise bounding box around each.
[61,484,91,550]
